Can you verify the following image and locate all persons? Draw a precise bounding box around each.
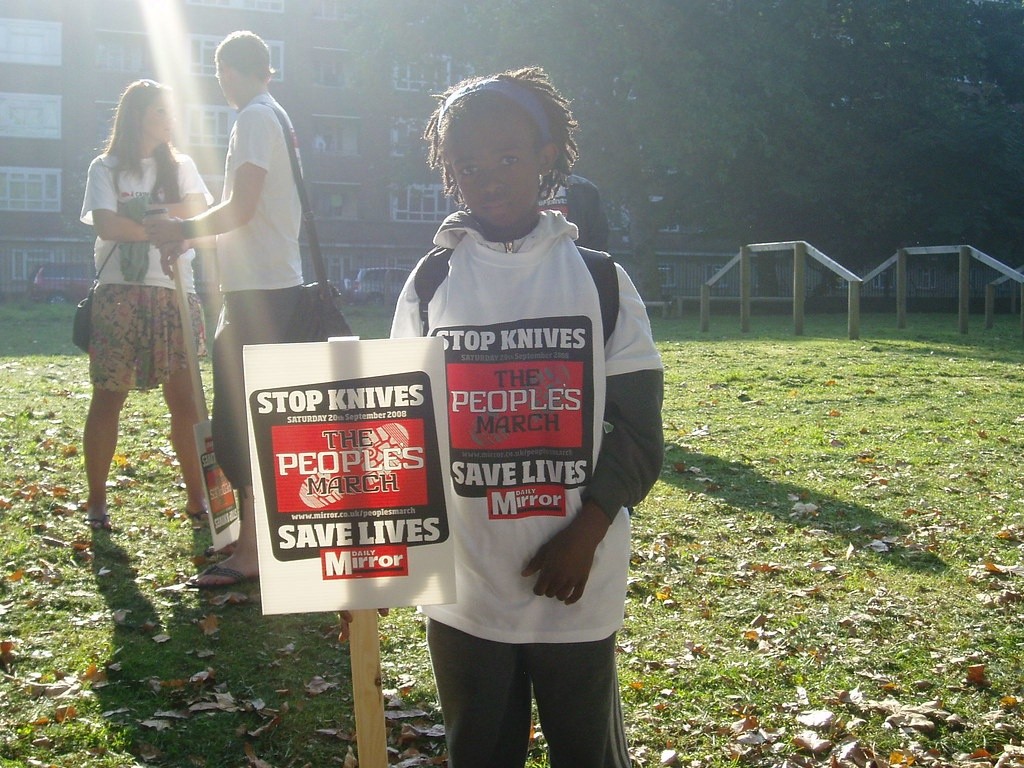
[83,79,215,529]
[143,31,307,587]
[389,67,664,768]
[538,174,609,252]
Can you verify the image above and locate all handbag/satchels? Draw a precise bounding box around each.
[286,278,356,343]
[72,287,94,353]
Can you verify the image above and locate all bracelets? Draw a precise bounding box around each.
[182,219,198,239]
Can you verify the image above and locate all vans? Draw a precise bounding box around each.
[33,262,97,306]
[355,267,415,307]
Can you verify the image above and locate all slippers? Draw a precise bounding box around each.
[185,564,259,588]
[83,513,113,531]
[186,506,209,521]
[205,542,238,557]
